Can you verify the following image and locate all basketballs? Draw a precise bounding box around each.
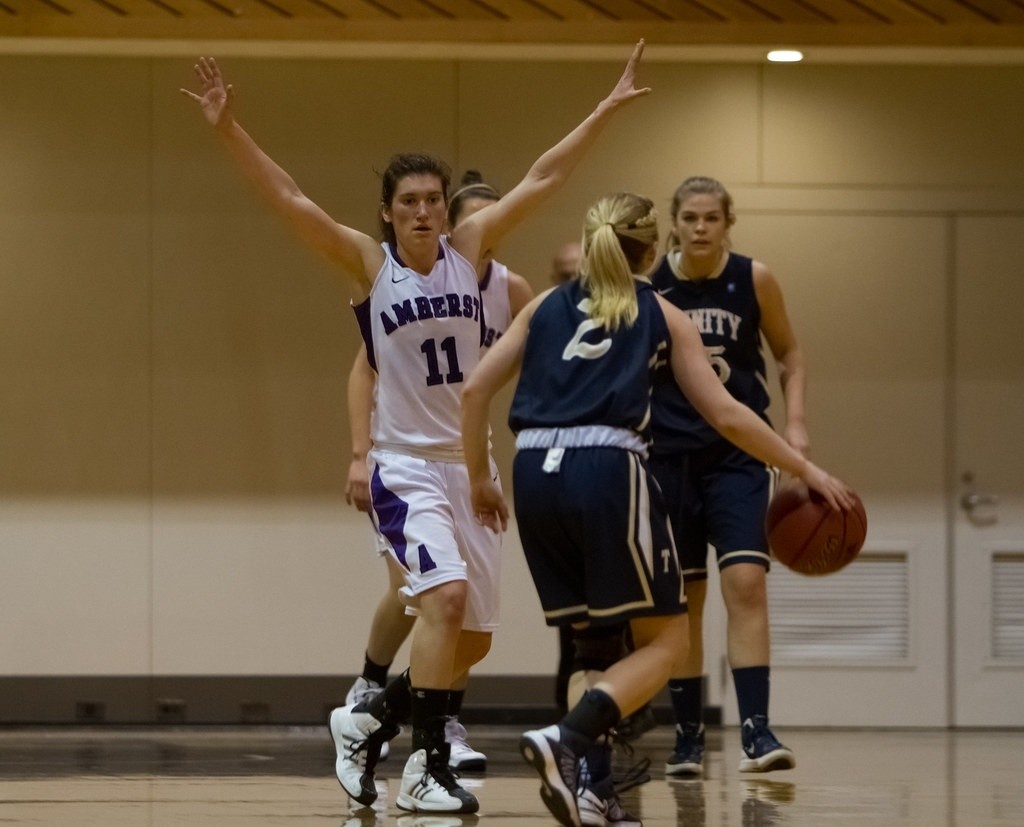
[764,479,867,576]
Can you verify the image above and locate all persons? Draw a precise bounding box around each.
[460,173,853,827]
[344,169,531,773]
[180,37,656,817]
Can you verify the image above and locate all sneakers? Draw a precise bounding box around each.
[444,715,487,773]
[328,695,400,807]
[396,741,479,814]
[664,722,706,774]
[738,714,796,772]
[578,757,651,827]
[519,724,590,827]
[345,675,389,762]
